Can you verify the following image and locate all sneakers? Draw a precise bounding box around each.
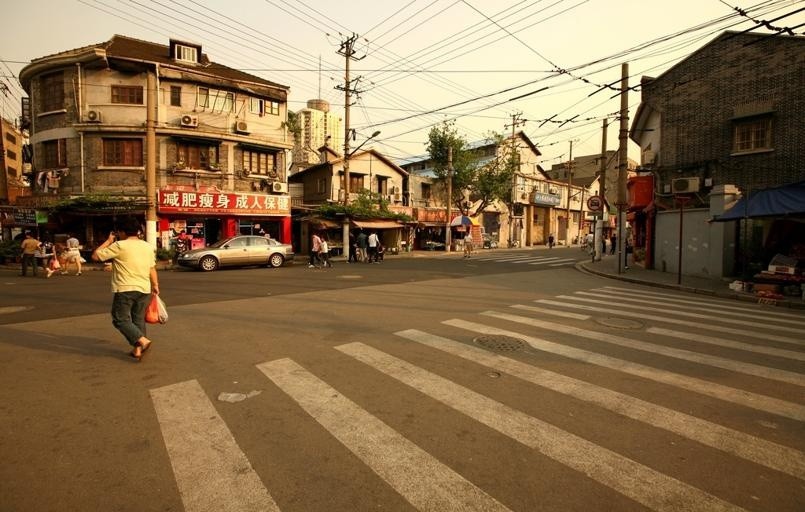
[61,271,68,274]
[308,264,315,268]
[323,261,326,267]
[47,270,53,277]
[329,264,332,268]
[346,257,384,265]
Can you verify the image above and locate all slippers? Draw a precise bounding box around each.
[130,341,152,359]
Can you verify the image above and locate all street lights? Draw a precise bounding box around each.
[342,132,381,262]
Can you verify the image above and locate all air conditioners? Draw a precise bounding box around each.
[393,195,402,201]
[271,182,287,193]
[181,114,198,127]
[641,151,654,165]
[82,110,104,123]
[672,177,700,193]
[393,186,402,195]
[237,120,254,133]
[551,187,558,193]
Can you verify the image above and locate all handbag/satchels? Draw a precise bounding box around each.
[376,241,380,247]
[50,259,61,271]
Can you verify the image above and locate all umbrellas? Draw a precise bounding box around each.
[451,214,481,239]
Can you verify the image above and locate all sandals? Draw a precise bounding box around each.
[76,272,82,276]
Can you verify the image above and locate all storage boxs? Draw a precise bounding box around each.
[768,253,796,275]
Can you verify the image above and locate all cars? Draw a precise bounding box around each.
[177,234,295,271]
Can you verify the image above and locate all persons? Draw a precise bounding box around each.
[548,233,554,249]
[575,231,629,269]
[463,231,473,258]
[90,217,161,360]
[308,229,384,269]
[172,229,193,250]
[18,231,90,277]
